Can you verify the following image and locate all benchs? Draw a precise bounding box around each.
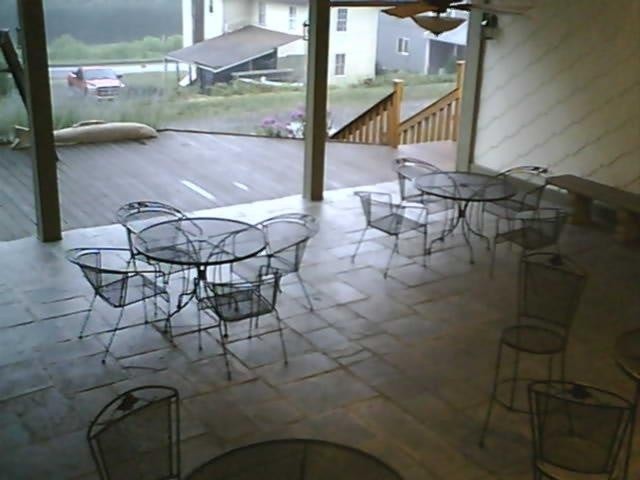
[546,175,638,240]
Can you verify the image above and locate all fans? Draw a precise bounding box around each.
[331,0,535,37]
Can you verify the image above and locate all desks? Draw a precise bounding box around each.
[417,172,519,265]
[181,437,408,479]
[616,329,640,480]
[134,218,269,353]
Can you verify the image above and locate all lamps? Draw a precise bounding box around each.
[413,0,468,35]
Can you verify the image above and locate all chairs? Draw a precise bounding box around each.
[476,258,587,448]
[66,247,173,363]
[227,214,320,327]
[489,207,567,275]
[86,386,181,479]
[117,201,204,314]
[350,191,430,279]
[393,158,460,242]
[530,378,635,480]
[194,265,288,380]
[481,166,554,250]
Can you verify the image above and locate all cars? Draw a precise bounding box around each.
[67,65,127,101]
